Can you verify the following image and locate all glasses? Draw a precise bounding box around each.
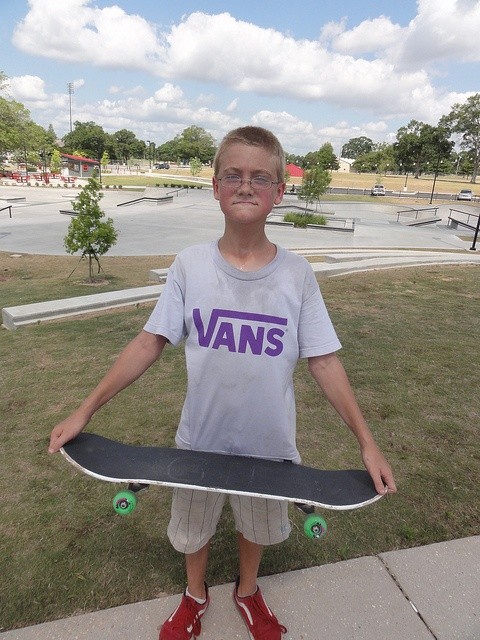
[215,175,281,192]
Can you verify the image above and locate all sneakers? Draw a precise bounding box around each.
[159,585,210,638]
[232,580,288,639]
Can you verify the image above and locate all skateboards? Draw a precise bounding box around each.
[59,432,387,539]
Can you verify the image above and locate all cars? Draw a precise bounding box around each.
[458,190,471,201]
[372,184,385,195]
[156,164,169,169]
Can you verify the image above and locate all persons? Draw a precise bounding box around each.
[290,184,295,192]
[48,126,396,640]
[371,187,374,195]
